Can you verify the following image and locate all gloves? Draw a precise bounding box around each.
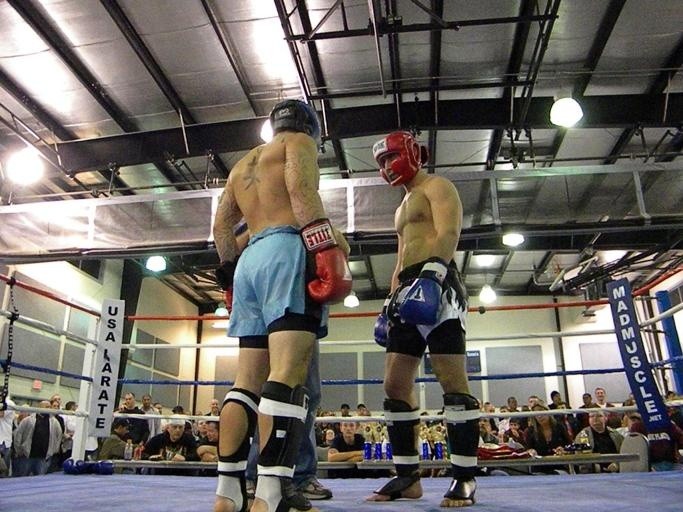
[306,245,354,307]
[224,285,232,315]
[397,273,448,326]
[371,291,394,347]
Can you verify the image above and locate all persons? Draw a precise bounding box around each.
[364,131,483,508]
[213,99,353,511]
[1,387,683,476]
[234,220,351,501]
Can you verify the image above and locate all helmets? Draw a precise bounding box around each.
[372,130,424,187]
[267,97,323,155]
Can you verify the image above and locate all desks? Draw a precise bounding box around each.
[102,456,358,475]
[356,454,640,478]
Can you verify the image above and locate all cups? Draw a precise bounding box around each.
[160,447,173,460]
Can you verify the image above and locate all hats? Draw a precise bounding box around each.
[628,412,641,420]
[357,403,366,409]
[340,403,351,409]
[167,412,186,428]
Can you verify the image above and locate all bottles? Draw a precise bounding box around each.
[123,438,144,460]
[508,437,513,447]
[363,439,444,462]
[578,430,589,444]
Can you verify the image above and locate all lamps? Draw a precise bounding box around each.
[550,80,584,126]
[478,272,496,304]
[344,290,359,307]
[214,292,230,317]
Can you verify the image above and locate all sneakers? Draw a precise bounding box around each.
[299,475,333,500]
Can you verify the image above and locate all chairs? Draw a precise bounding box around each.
[618,432,653,473]
